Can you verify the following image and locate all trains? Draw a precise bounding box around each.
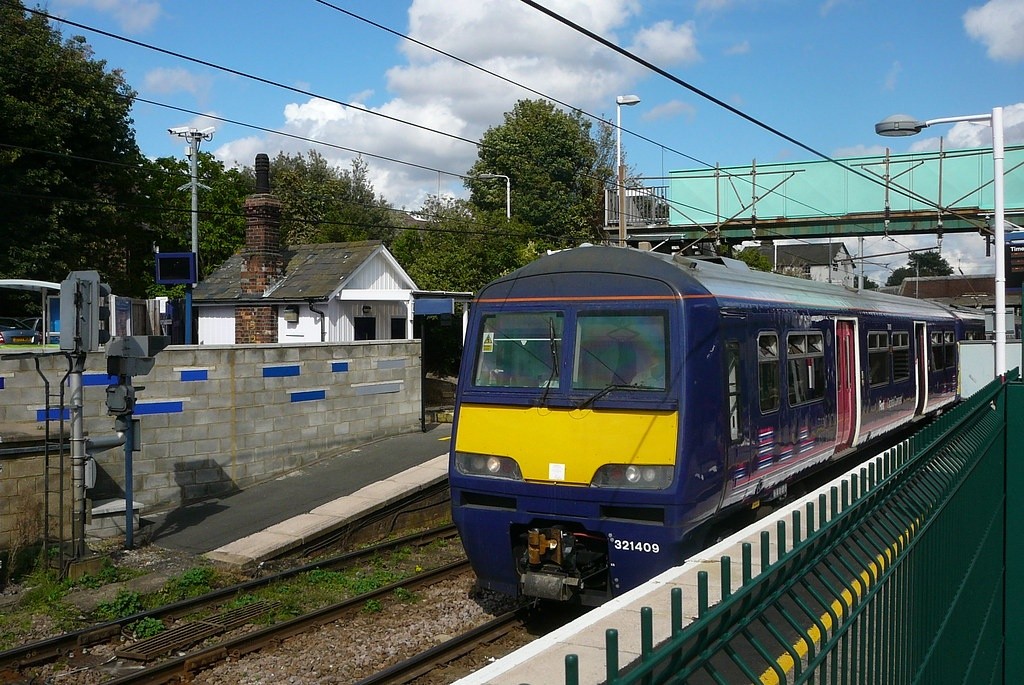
[448,241,985,617]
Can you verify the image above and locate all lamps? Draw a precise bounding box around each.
[363,305,372,313]
[284,304,299,323]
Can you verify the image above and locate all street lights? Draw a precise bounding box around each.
[478,172,510,223]
[615,95,641,196]
[875,106,1005,376]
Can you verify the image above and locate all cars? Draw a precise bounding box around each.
[0,317,47,345]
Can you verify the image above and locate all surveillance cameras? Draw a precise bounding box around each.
[168,127,189,135]
[190,127,216,142]
